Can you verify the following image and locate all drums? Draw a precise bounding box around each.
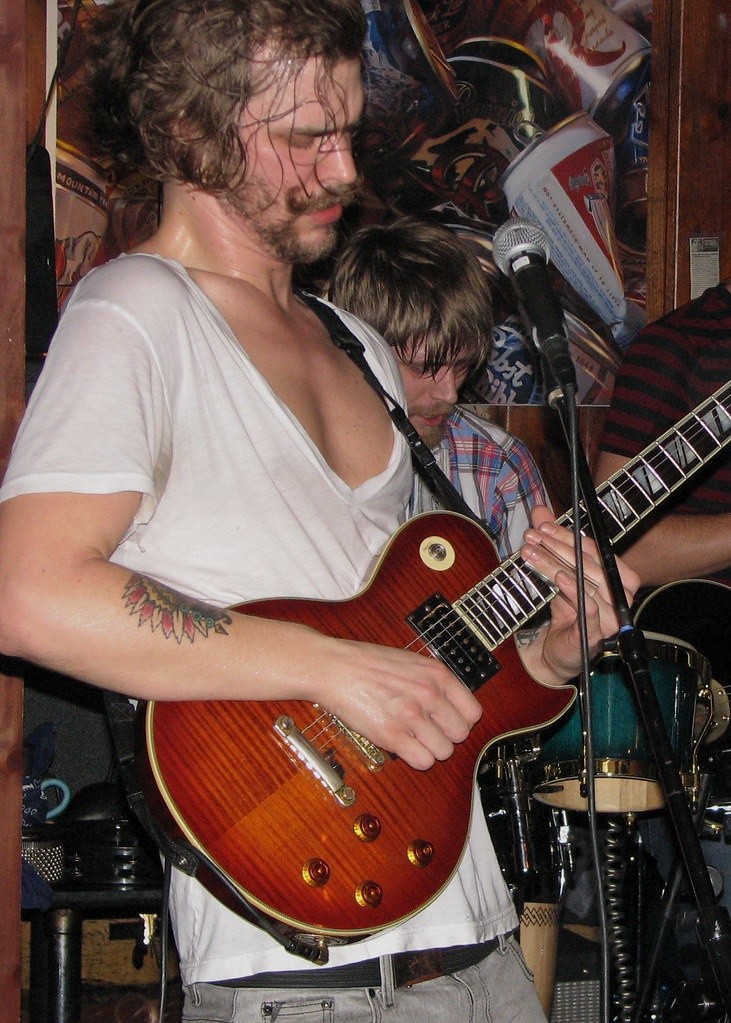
[528,629,706,818]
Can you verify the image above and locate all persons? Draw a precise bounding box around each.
[319,220,556,569]
[0,3,636,1023]
[595,259,731,677]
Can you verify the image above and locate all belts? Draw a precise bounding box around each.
[202,927,520,988]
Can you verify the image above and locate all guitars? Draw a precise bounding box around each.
[633,576,731,816]
[146,380,729,938]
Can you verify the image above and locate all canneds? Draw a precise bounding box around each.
[356,0,653,406]
[54,1,162,322]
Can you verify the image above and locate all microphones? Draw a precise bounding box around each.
[492,216,580,394]
